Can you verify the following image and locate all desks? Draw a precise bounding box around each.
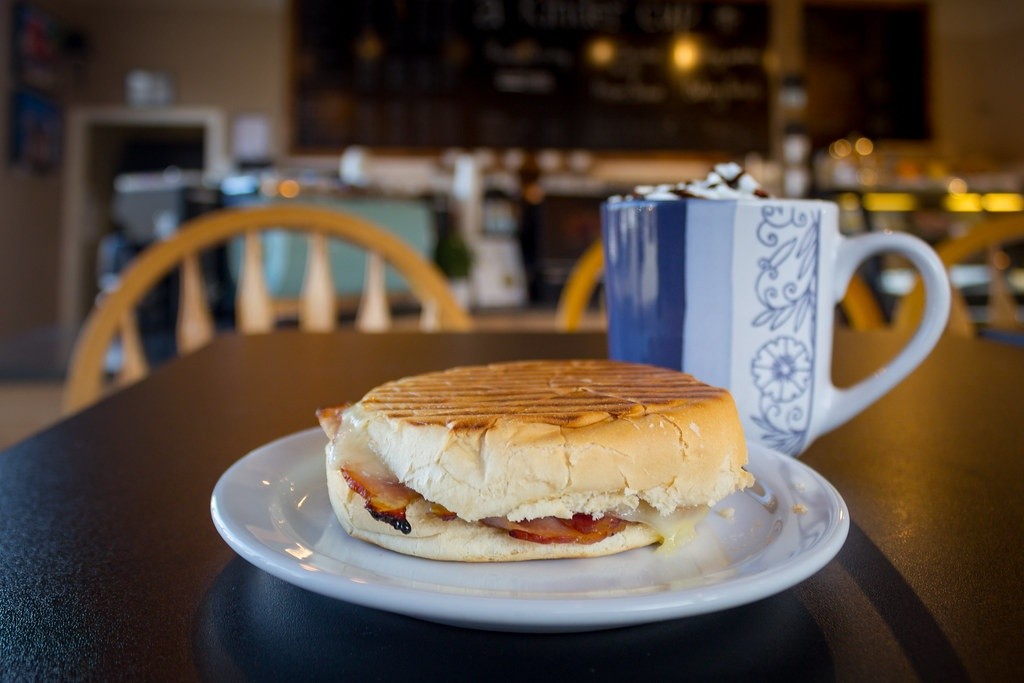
[0,336,1024,683]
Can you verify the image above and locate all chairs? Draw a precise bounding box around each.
[57,202,472,414]
[558,224,886,330]
[894,214,1024,336]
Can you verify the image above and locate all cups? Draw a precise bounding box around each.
[601,197,951,457]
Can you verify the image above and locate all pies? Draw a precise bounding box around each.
[316,359,748,561]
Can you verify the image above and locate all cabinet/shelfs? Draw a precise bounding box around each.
[58,106,230,370]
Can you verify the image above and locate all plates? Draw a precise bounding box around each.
[209,428,852,634]
